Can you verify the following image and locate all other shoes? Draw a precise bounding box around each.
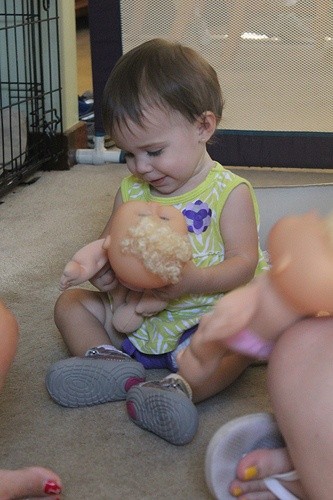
[45,344,146,408]
[126,377,197,444]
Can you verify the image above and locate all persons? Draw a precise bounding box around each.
[177,210,333,403]
[43,37,272,445]
[57,199,193,334]
[204,315,333,500]
[0,302,62,500]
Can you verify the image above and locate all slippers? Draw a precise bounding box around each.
[204,411,301,500]
